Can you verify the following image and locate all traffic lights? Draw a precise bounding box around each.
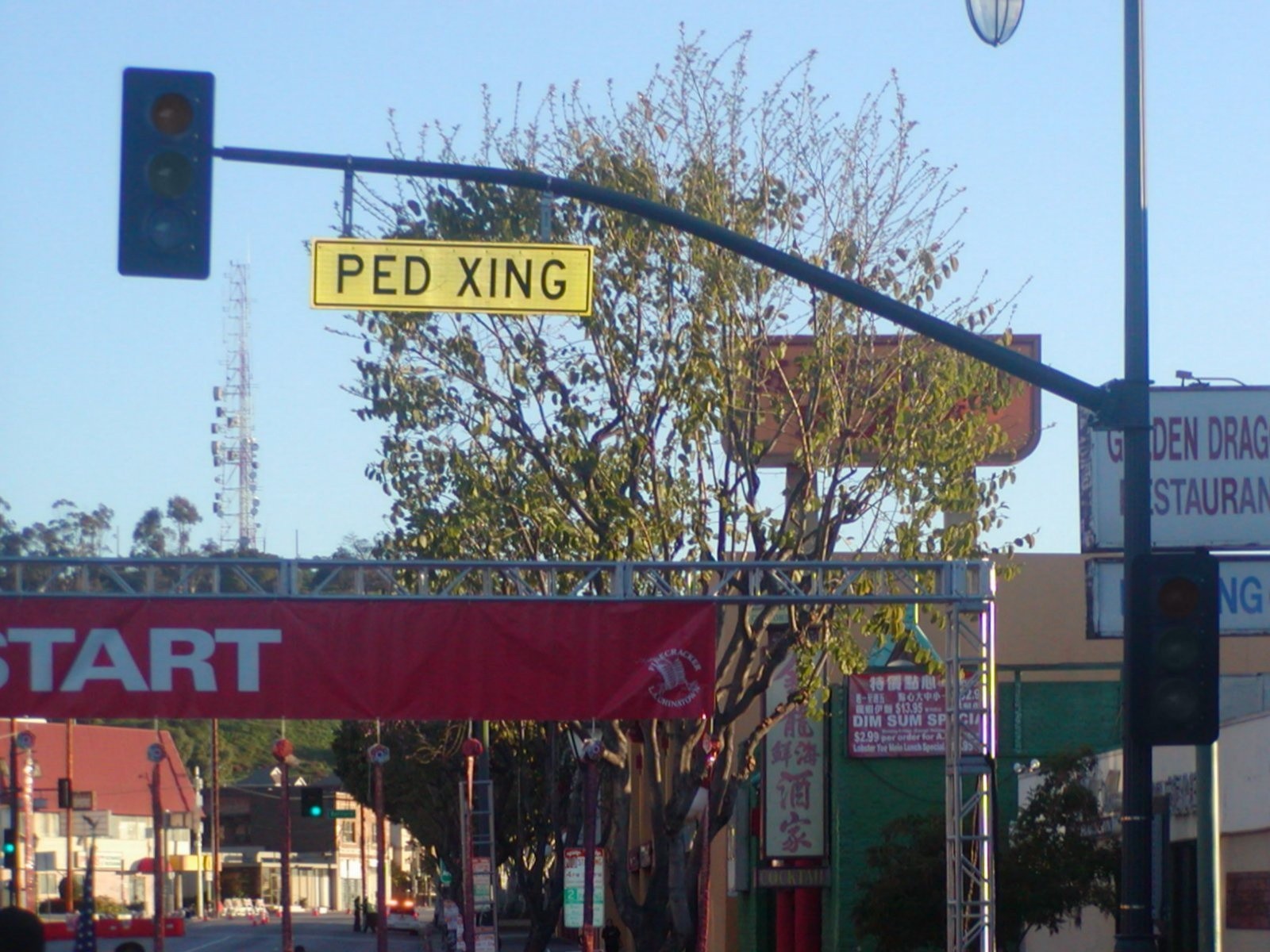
[301,785,324,816]
[119,65,214,279]
[1130,551,1221,744]
[3,827,16,869]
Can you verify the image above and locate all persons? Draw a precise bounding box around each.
[353,895,362,932]
[601,918,622,952]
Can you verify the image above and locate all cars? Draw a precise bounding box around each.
[386,902,420,932]
